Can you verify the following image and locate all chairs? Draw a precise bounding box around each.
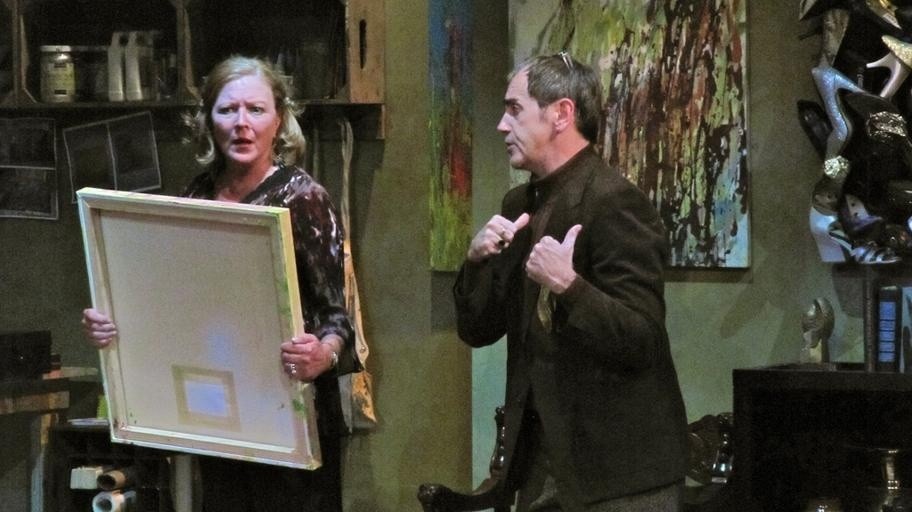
[415,405,732,512]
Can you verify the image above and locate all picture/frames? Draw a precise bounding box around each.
[76,186,322,472]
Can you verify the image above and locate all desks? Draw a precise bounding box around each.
[0,377,70,512]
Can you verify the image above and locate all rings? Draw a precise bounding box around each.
[500,229,506,236]
[497,239,506,248]
[288,362,297,377]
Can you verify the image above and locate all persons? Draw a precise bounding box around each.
[450,51,697,511]
[79,52,362,510]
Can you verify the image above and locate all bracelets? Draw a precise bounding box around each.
[320,341,339,370]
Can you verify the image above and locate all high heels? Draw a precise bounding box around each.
[795,0,912,266]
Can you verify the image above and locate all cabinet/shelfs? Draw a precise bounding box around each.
[732,362,911,512]
[0,0,386,140]
